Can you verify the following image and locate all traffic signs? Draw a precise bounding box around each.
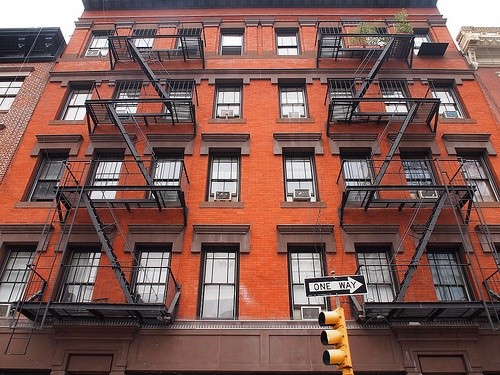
[303,274,368,298]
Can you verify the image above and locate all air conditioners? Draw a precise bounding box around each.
[84,49,102,58]
[116,110,130,119]
[418,190,438,199]
[293,188,311,201]
[442,110,461,119]
[301,306,322,320]
[0,304,13,317]
[219,109,234,117]
[288,111,300,118]
[213,190,232,201]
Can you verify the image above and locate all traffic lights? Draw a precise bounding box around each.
[318,306,352,370]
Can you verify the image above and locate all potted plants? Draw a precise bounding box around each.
[394,22,413,40]
[347,21,380,48]
